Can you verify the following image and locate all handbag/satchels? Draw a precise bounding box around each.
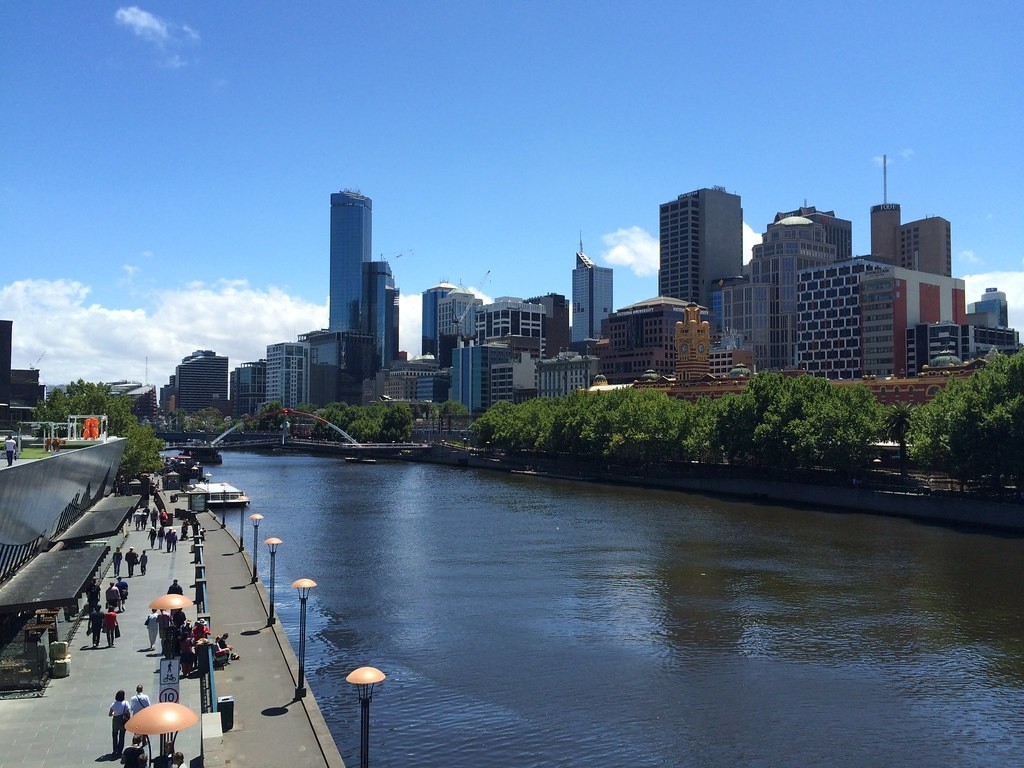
[144,616,150,625]
[120,589,127,600]
[122,713,129,723]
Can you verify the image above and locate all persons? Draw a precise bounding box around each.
[125,547,137,578]
[3,435,16,466]
[137,551,147,576]
[147,527,178,552]
[113,547,122,577]
[134,504,168,531]
[201,527,206,541]
[144,608,239,678]
[109,684,189,768]
[181,518,190,538]
[167,579,183,615]
[85,576,129,648]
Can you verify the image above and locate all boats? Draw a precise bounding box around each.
[345,456,377,465]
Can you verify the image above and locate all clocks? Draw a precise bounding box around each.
[681,344,689,353]
[698,343,704,353]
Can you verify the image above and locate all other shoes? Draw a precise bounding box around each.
[149,645,155,650]
[122,607,125,611]
[118,610,123,612]
[136,528,139,531]
[93,642,100,647]
[109,643,115,647]
[112,752,122,758]
[142,572,146,576]
[129,574,134,578]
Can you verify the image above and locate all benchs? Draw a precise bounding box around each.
[889,475,931,496]
[213,639,228,670]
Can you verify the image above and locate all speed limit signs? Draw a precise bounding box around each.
[159,688,179,703]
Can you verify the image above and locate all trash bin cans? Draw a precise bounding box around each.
[217,696,234,730]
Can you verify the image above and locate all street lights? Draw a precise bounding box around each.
[345,666,387,768]
[265,537,282,624]
[291,578,318,697]
[149,594,195,756]
[236,496,249,552]
[219,482,229,529]
[248,513,265,582]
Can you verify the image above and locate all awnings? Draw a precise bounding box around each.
[0,545,111,613]
[56,491,143,542]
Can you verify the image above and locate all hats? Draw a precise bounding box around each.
[186,619,191,624]
[151,527,155,529]
[197,618,207,625]
[169,527,172,530]
[129,546,134,549]
[173,530,177,533]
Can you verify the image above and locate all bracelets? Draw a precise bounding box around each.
[4,450,5,451]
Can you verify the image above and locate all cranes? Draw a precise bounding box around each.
[450,269,491,335]
[29,350,48,369]
